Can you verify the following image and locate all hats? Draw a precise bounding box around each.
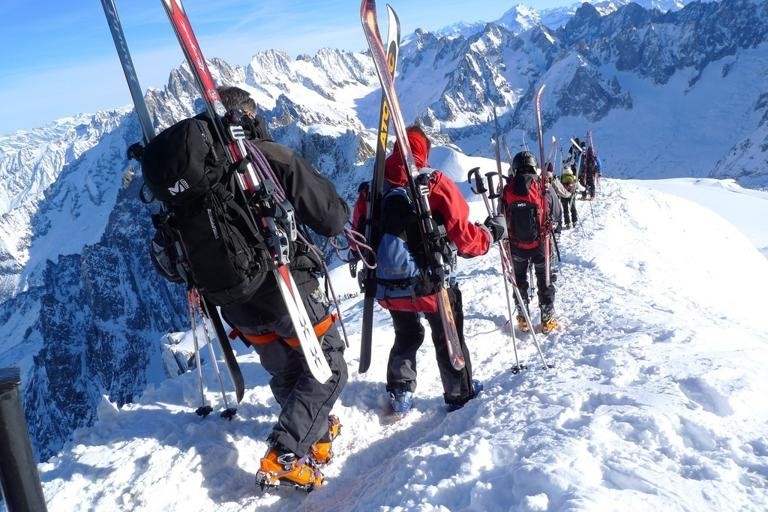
[545,162,553,171]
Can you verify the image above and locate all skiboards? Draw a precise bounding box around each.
[487,83,598,289]
[101,0,334,402]
[359,0,466,371]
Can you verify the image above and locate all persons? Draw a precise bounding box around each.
[497,150,563,337]
[150,84,355,488]
[347,122,511,415]
[539,136,602,236]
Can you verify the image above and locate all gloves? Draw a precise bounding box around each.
[485,212,507,242]
[586,185,591,189]
[570,188,577,196]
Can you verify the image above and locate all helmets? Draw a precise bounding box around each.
[513,152,537,169]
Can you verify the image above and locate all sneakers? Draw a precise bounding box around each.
[447,379,483,411]
[581,196,594,200]
[390,392,413,412]
[566,222,577,227]
[308,415,340,464]
[256,444,324,490]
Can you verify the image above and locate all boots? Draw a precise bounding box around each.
[540,304,557,334]
[516,303,531,332]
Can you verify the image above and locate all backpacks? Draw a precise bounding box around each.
[141,112,276,309]
[561,174,576,192]
[503,176,544,249]
[365,168,439,298]
